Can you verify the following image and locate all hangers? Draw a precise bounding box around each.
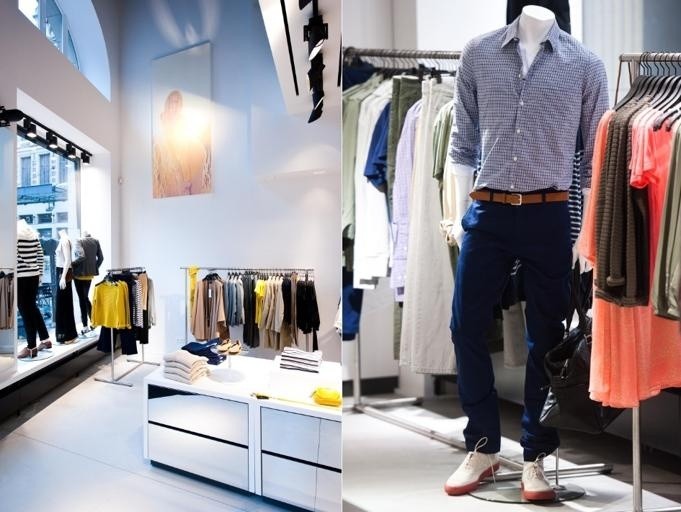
[203,268,221,282]
[105,268,141,282]
[610,52,681,131]
[343,47,460,82]
[227,266,312,282]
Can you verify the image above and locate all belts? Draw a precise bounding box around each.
[470,190,572,205]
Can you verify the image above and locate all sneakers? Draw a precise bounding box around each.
[521,458,556,501]
[229,339,241,353]
[38,342,52,350]
[17,347,38,358]
[217,338,232,352]
[444,452,501,497]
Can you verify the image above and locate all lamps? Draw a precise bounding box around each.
[82,152,89,165]
[46,131,58,149]
[307,97,324,122]
[298,2,310,11]
[307,50,325,109]
[23,119,37,138]
[304,1,329,61]
[66,144,77,159]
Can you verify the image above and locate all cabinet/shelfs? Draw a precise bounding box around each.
[143,351,341,512]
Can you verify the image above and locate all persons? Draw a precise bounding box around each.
[53,229,78,345]
[16,219,52,359]
[72,230,104,335]
[447,5,609,504]
